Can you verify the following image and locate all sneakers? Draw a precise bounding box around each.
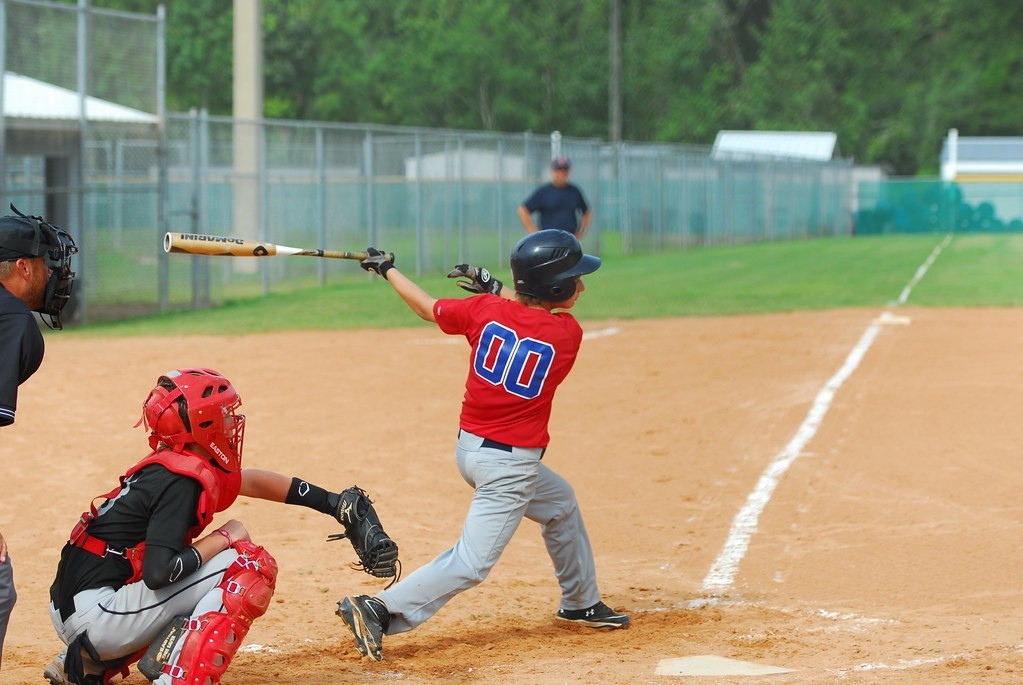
[336,595,391,662]
[556,600,628,628]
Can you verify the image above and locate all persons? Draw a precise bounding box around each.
[0,214,80,665]
[518,154,591,314]
[43,368,398,685]
[336,229,628,662]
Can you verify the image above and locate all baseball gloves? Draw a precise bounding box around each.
[323,484,403,590]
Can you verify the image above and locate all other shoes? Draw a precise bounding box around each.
[44,646,102,685]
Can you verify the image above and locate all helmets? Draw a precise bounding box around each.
[136,368,245,474]
[510,228,602,302]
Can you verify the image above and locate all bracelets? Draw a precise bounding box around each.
[214,529,231,547]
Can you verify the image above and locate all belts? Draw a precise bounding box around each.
[58,601,75,620]
[456,430,545,460]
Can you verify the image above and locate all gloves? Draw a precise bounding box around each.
[360,247,394,280]
[447,264,503,296]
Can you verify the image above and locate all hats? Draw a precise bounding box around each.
[0,215,50,259]
[553,158,570,169]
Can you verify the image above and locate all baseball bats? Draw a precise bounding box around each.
[163,231,395,264]
